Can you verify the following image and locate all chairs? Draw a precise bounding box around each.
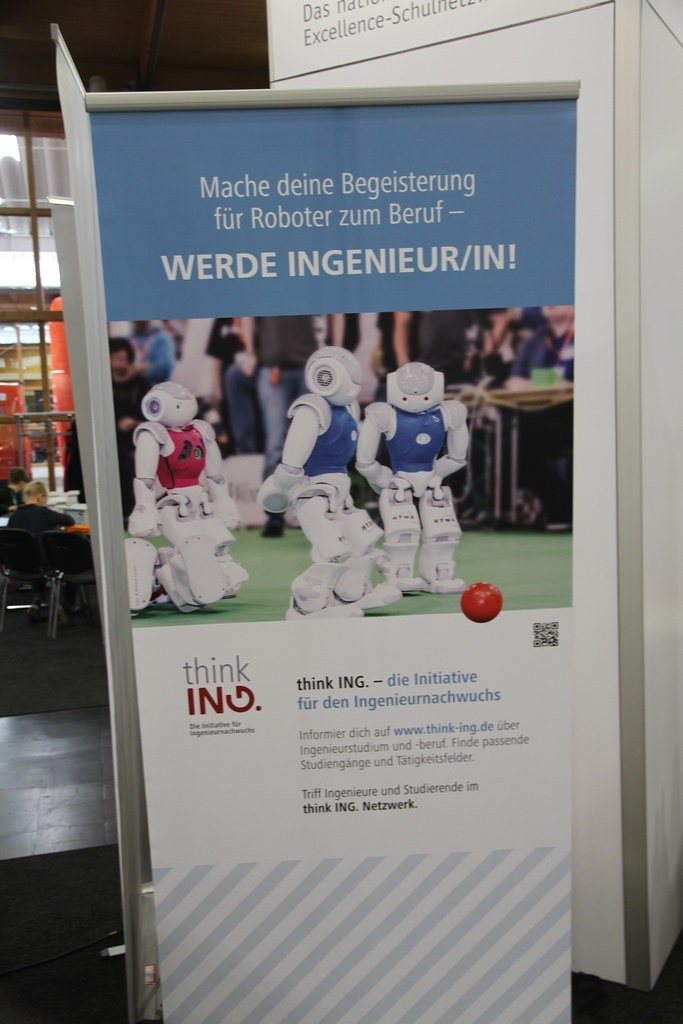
[0,528,55,639]
[41,529,99,637]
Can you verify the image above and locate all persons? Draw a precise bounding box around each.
[64,420,86,505]
[108,308,573,530]
[8,481,91,623]
[130,346,470,617]
[0,468,28,514]
